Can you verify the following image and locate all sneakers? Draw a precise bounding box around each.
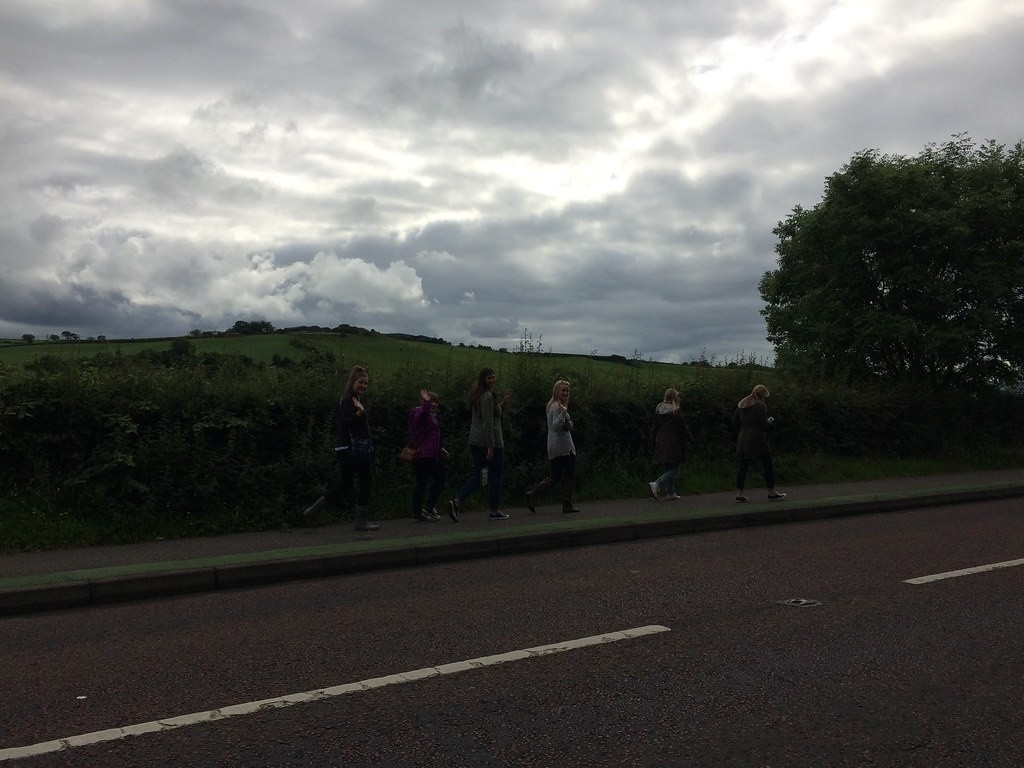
[667,492,681,501]
[735,495,751,503]
[411,505,441,522]
[448,499,460,523]
[768,491,787,501]
[489,511,510,520]
[648,482,660,501]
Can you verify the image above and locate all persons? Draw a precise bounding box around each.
[647,388,693,501]
[405,388,445,523]
[448,367,512,521]
[301,365,381,532]
[520,379,581,514]
[731,385,787,502]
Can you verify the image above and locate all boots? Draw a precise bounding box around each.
[303,496,328,531]
[562,496,581,513]
[523,487,539,514]
[353,504,380,531]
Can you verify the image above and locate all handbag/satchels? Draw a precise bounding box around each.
[399,446,418,462]
[350,438,374,459]
